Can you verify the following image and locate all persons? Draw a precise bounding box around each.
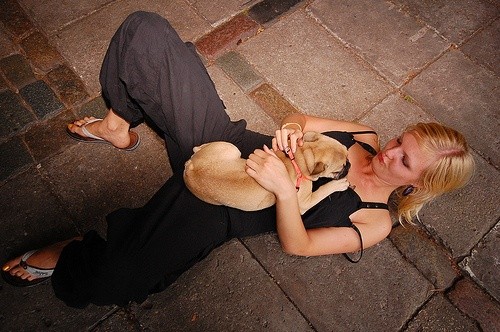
[0,10,477,309]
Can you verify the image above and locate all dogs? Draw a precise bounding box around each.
[183,130,351,215]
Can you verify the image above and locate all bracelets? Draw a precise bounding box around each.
[281,120,305,133]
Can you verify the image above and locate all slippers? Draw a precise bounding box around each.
[66,117,141,151]
[0,232,84,288]
